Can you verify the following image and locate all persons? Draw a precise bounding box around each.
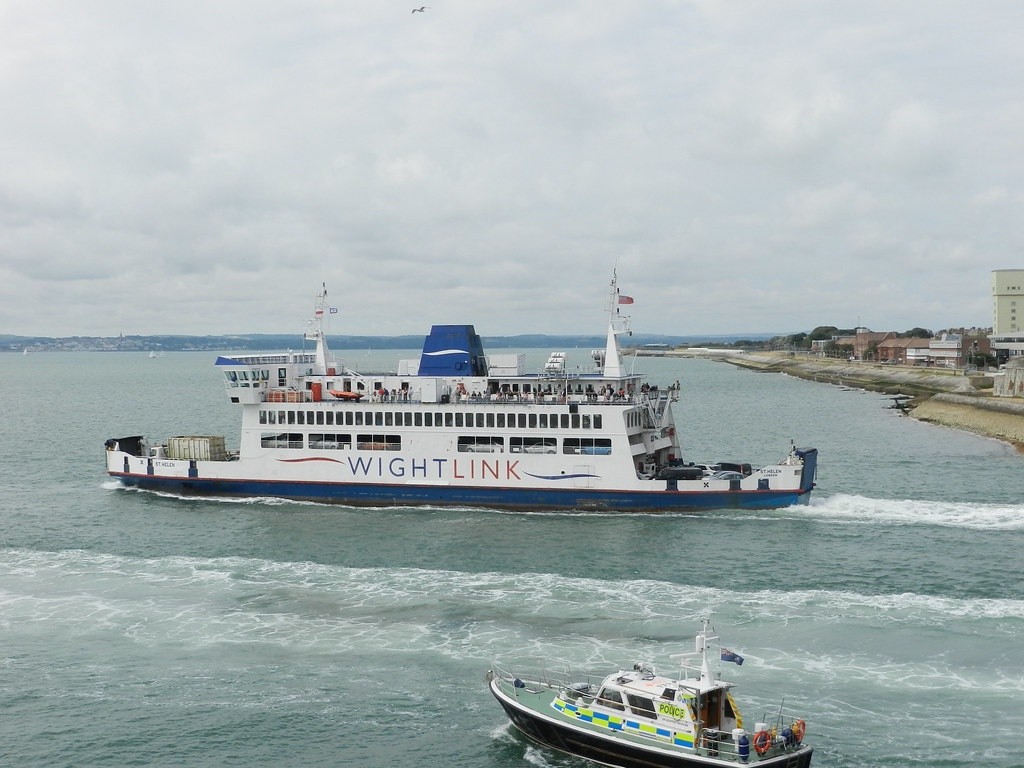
[450,383,680,404]
[374,386,413,403]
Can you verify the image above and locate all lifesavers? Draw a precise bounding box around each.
[753,731,771,753]
[795,719,806,741]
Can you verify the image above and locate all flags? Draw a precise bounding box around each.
[618,295,633,305]
[720,647,745,666]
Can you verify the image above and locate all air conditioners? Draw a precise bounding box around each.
[150,447,163,458]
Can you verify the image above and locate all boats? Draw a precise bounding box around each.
[485,616,818,768]
[101,264,821,515]
[329,389,365,399]
